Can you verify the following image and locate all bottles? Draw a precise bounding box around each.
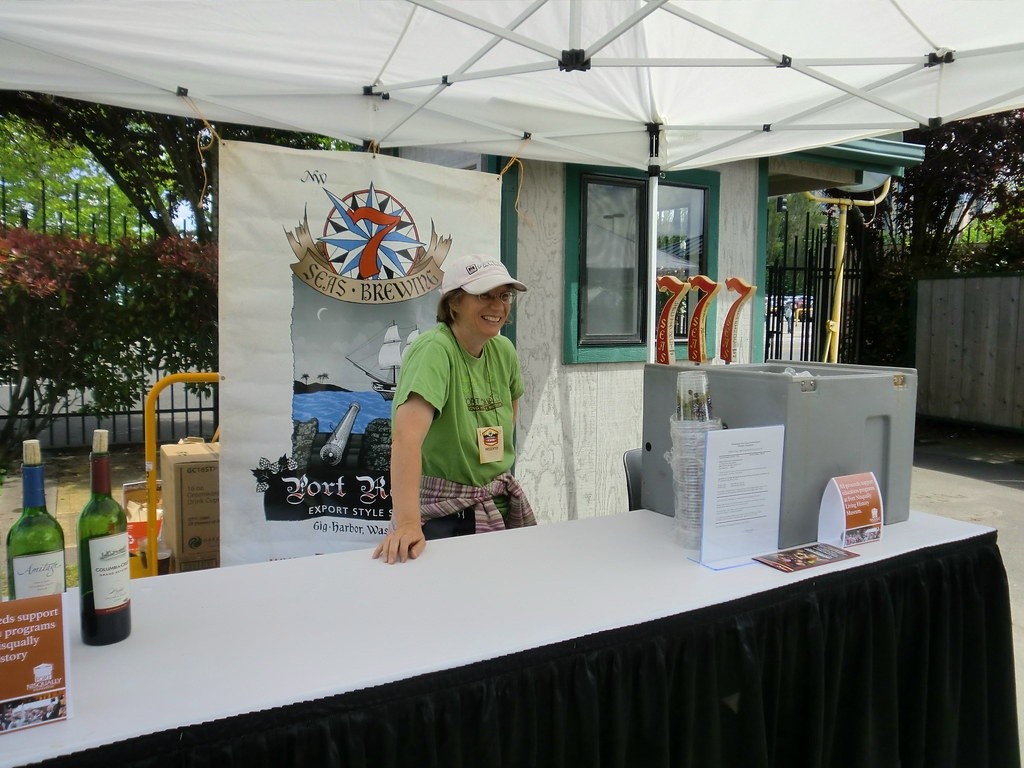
[7,440,67,602]
[78,430,131,645]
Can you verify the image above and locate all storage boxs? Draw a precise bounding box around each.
[160,441,219,573]
[641,358,919,551]
[122,481,163,554]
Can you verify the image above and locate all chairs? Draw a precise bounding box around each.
[622,448,642,511]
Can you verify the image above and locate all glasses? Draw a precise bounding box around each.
[464,292,517,305]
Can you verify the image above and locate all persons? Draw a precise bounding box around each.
[372,254,528,564]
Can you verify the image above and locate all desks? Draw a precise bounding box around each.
[0,506,1001,768]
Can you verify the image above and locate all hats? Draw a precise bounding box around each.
[439,254,528,297]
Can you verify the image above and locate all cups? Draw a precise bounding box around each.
[674,371,718,550]
